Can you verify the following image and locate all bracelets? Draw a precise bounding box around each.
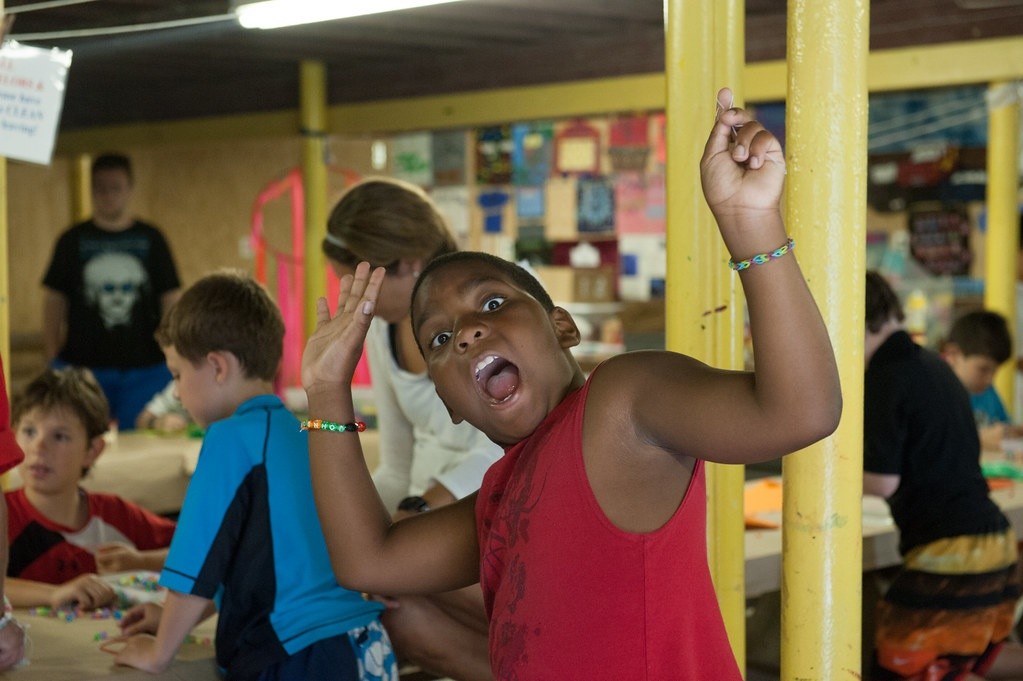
[728,238,796,270]
[300,420,365,433]
[0,595,13,631]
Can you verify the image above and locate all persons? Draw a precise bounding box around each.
[38,146,186,436]
[299,86,843,681]
[864,262,1023,681]
[0,365,178,671]
[113,273,400,681]
